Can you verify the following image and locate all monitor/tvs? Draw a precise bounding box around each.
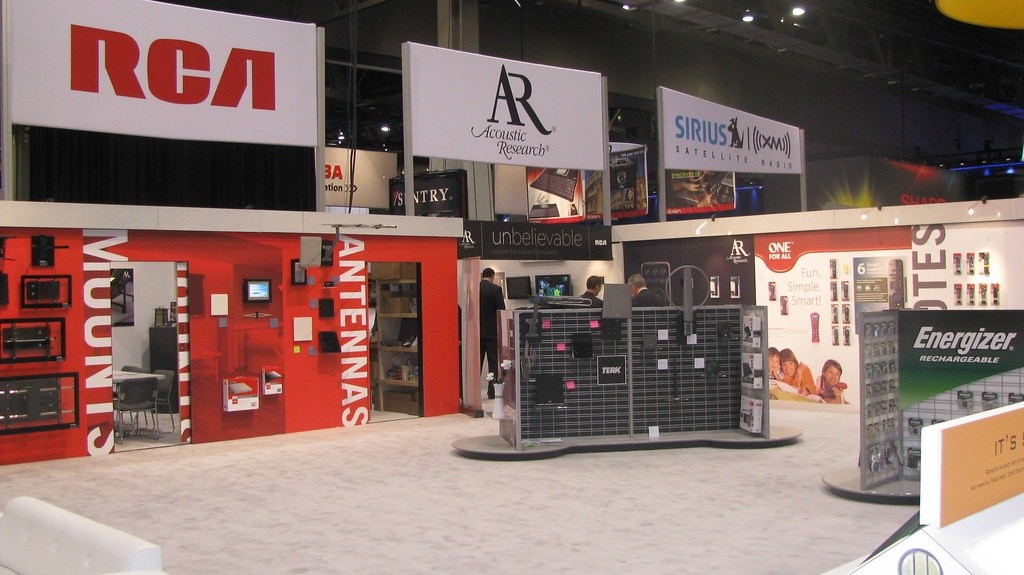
[506,276,532,300]
[535,274,572,296]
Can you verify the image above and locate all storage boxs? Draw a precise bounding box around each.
[379,297,411,315]
[382,390,419,416]
[370,262,417,281]
[222,376,258,412]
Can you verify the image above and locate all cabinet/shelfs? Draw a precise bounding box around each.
[148,326,180,413]
[375,280,419,417]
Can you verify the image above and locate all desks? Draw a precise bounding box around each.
[112,370,166,384]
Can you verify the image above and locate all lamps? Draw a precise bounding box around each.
[337,127,345,140]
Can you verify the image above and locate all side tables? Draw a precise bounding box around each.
[262,365,281,395]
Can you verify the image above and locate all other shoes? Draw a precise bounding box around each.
[487,389,495,399]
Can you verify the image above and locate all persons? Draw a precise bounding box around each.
[779,348,816,397]
[578,275,603,308]
[480,267,506,400]
[628,273,664,307]
[767,346,780,380]
[815,358,844,404]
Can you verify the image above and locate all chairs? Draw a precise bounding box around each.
[112,365,175,445]
